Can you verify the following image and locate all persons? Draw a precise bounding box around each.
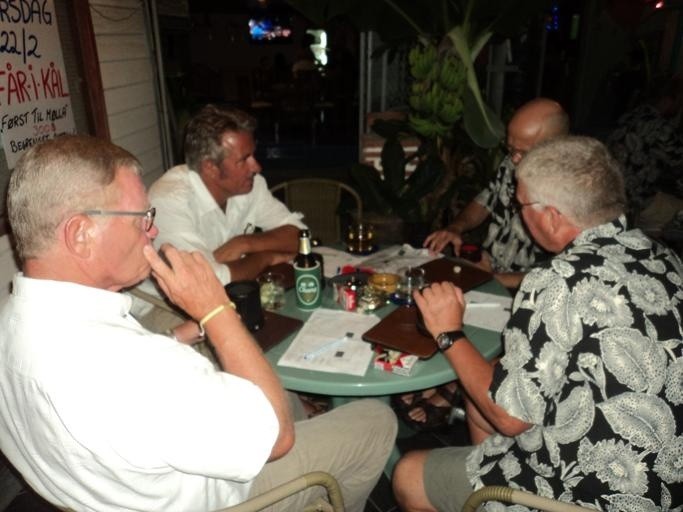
[397,98,573,433]
[0,130,402,510]
[392,135,683,512]
[114,102,313,362]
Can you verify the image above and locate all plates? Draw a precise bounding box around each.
[327,270,372,288]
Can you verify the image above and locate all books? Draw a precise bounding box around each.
[257,262,295,291]
[408,256,494,294]
[237,302,305,352]
[360,300,443,361]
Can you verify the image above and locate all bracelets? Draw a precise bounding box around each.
[196,299,238,337]
[163,325,181,347]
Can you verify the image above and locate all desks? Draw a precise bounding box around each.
[226,250,513,487]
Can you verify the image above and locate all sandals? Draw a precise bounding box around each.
[389,390,429,414]
[402,384,459,428]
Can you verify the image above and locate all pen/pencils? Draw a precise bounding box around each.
[304,335,347,361]
[463,302,505,308]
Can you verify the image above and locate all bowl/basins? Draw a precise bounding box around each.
[365,274,400,298]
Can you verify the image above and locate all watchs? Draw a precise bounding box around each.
[435,326,467,355]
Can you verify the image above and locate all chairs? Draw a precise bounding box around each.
[458,484,587,512]
[220,466,354,512]
[271,178,364,247]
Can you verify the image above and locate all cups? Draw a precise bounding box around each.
[224,280,266,334]
[460,241,481,265]
[395,267,426,303]
[345,222,377,254]
[256,271,285,311]
[293,252,325,291]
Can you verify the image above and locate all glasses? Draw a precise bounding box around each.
[81,206,157,232]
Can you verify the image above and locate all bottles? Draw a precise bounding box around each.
[292,228,322,311]
